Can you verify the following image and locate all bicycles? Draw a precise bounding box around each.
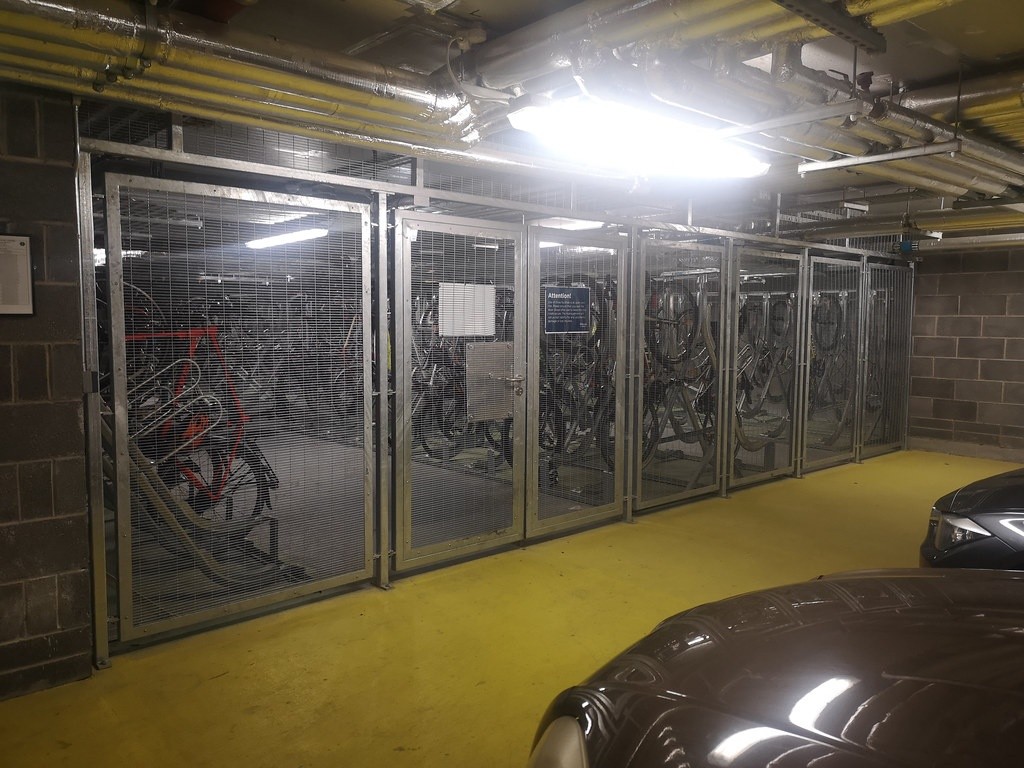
[99,269,851,545]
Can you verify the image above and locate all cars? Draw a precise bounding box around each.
[915,463,1024,571]
[524,562,1024,767]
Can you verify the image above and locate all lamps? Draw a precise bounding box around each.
[700,46,862,138]
[797,72,962,174]
